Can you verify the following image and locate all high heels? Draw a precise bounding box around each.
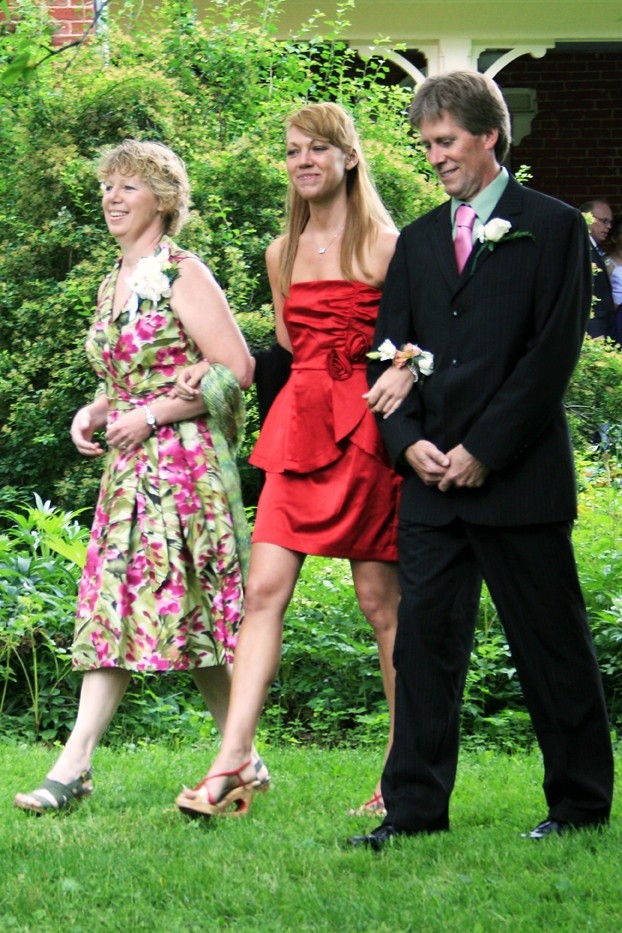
[14,772,92,813]
[174,764,253,817]
[253,759,271,791]
[358,793,387,818]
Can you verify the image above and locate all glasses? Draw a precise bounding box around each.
[596,217,614,224]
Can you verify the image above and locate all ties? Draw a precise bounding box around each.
[453,206,476,275]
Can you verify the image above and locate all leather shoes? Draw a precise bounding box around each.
[519,818,576,840]
[342,823,452,849]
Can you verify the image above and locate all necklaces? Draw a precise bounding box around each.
[307,226,343,254]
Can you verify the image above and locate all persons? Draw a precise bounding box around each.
[170,102,434,817]
[12,139,271,821]
[344,70,614,851]
[579,201,622,352]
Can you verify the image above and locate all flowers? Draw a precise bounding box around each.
[366,338,434,376]
[112,247,173,320]
[470,218,535,275]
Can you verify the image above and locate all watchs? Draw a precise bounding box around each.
[144,405,159,430]
